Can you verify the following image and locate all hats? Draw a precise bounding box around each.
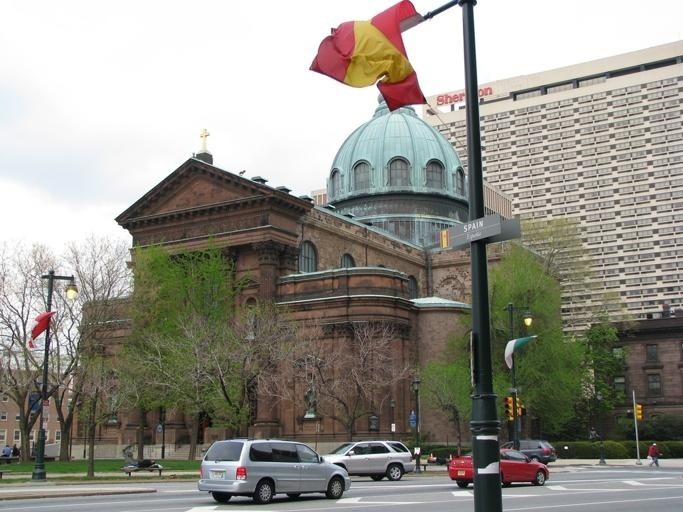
[198,439,415,504]
[447,438,558,489]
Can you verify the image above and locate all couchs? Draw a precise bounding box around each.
[504,396,522,420]
[626,403,644,420]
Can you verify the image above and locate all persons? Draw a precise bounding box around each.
[0,443,20,457]
[119,459,155,468]
[647,443,663,467]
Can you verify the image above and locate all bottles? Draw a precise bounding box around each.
[652,443,657,446]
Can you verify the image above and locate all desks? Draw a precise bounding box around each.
[390,398,395,440]
[502,302,534,450]
[411,375,423,473]
[31,267,78,480]
